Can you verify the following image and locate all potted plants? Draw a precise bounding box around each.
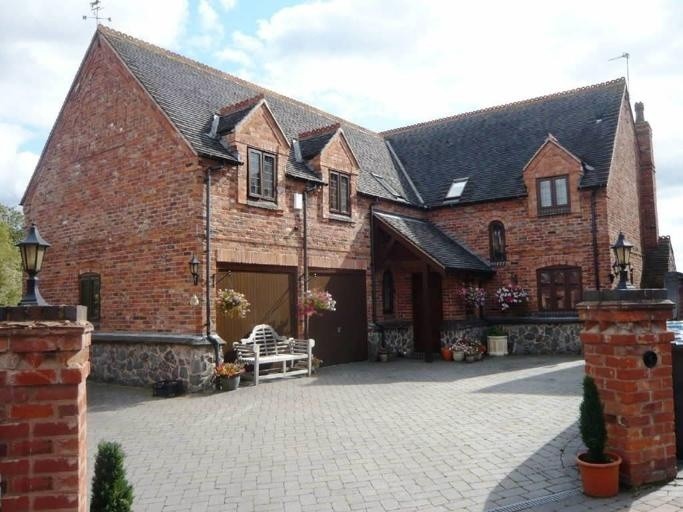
[576,373,623,498]
[439,325,511,362]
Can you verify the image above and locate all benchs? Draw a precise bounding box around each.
[232,324,315,386]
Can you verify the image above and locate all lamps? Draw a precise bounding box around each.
[609,231,636,289]
[15,221,52,306]
[188,252,202,287]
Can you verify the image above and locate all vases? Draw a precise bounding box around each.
[222,377,241,391]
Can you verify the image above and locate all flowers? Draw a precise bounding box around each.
[494,284,530,312]
[214,286,252,319]
[294,287,337,321]
[217,359,244,376]
[458,286,488,307]
